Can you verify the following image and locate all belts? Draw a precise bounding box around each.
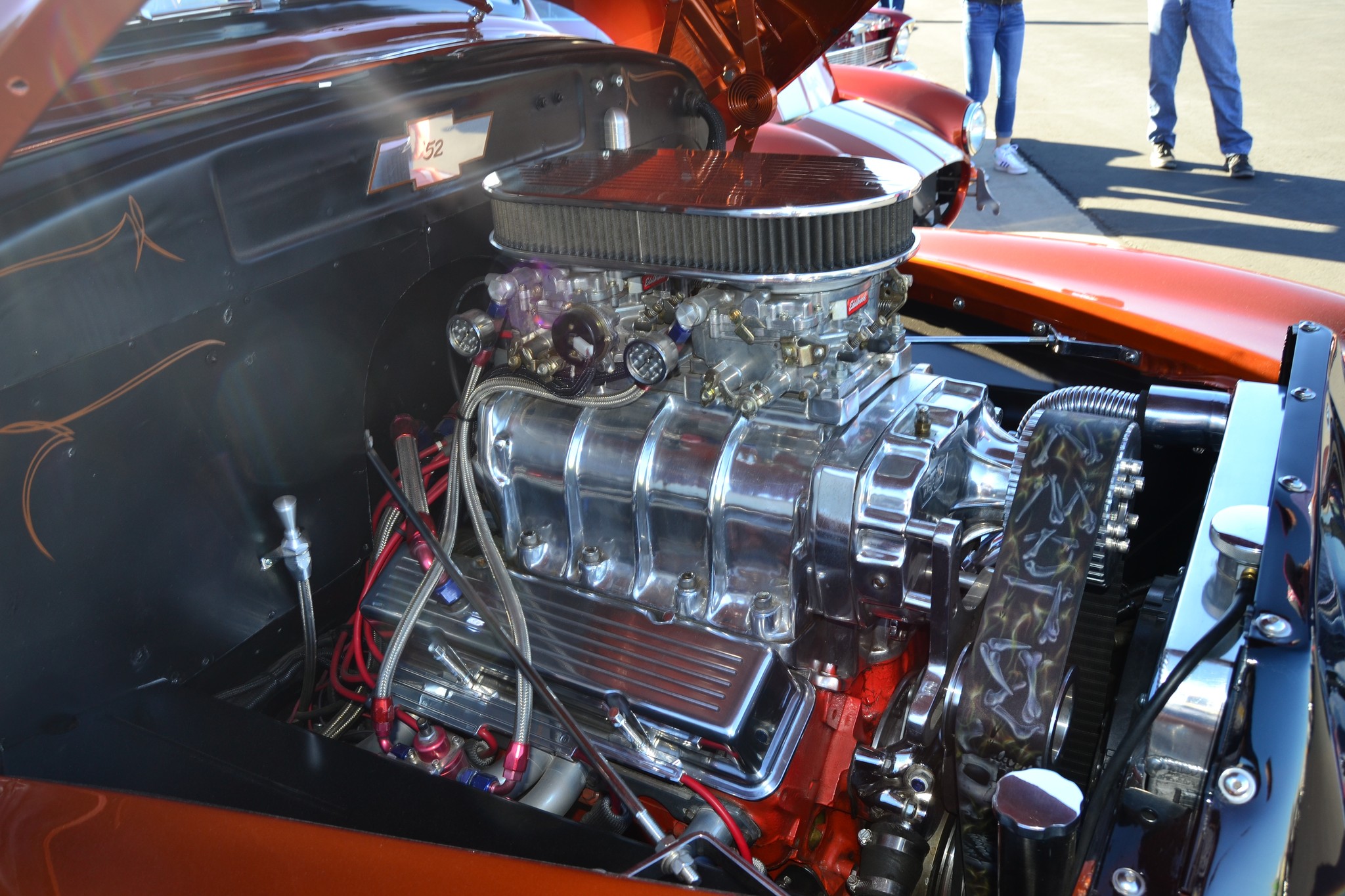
[968,0,1022,6]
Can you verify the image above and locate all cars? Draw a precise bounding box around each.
[419,1,999,227]
[0,0,1343,895]
[815,3,917,73]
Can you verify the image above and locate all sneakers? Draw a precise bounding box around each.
[993,143,1029,174]
[1150,140,1178,169]
[1223,153,1256,177]
[970,161,989,181]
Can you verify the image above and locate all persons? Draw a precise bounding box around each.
[878,0,906,12]
[962,0,1027,184]
[1145,0,1256,179]
